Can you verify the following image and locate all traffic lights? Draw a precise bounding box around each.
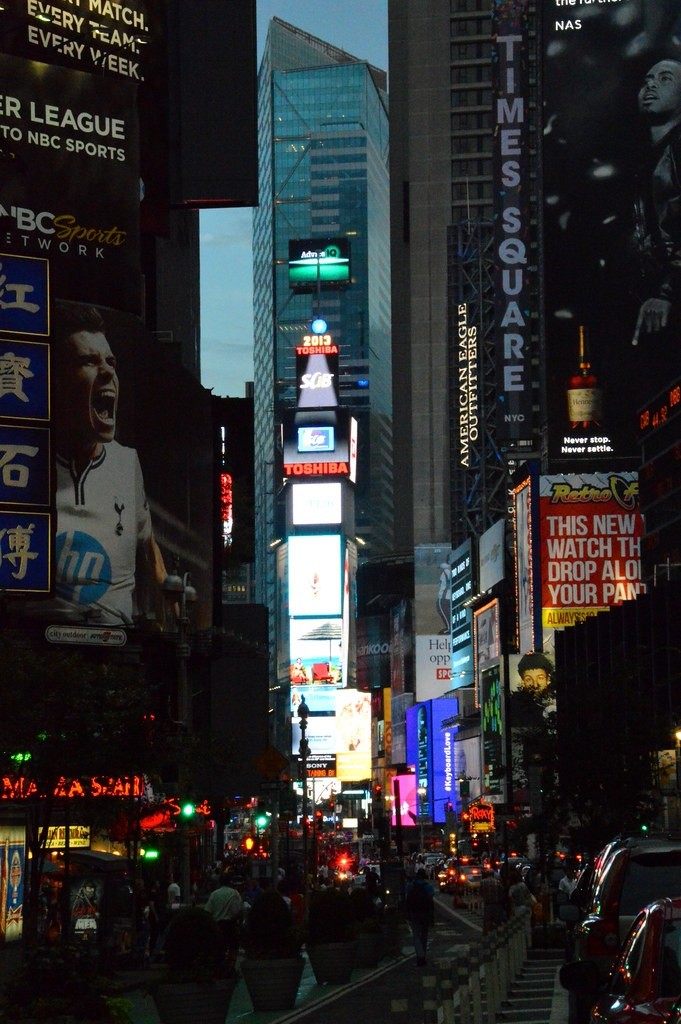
[640,810,650,833]
[461,807,470,824]
[143,837,161,874]
[374,786,382,802]
[179,786,195,822]
[316,810,323,829]
[254,801,269,830]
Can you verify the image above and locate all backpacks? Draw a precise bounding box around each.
[406,882,431,914]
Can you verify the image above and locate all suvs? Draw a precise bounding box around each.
[556,837,681,984]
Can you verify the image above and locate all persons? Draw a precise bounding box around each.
[10,819,588,978]
[12,302,188,635]
[517,652,553,692]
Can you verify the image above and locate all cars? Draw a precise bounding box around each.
[589,894,680,1024]
[320,838,622,940]
